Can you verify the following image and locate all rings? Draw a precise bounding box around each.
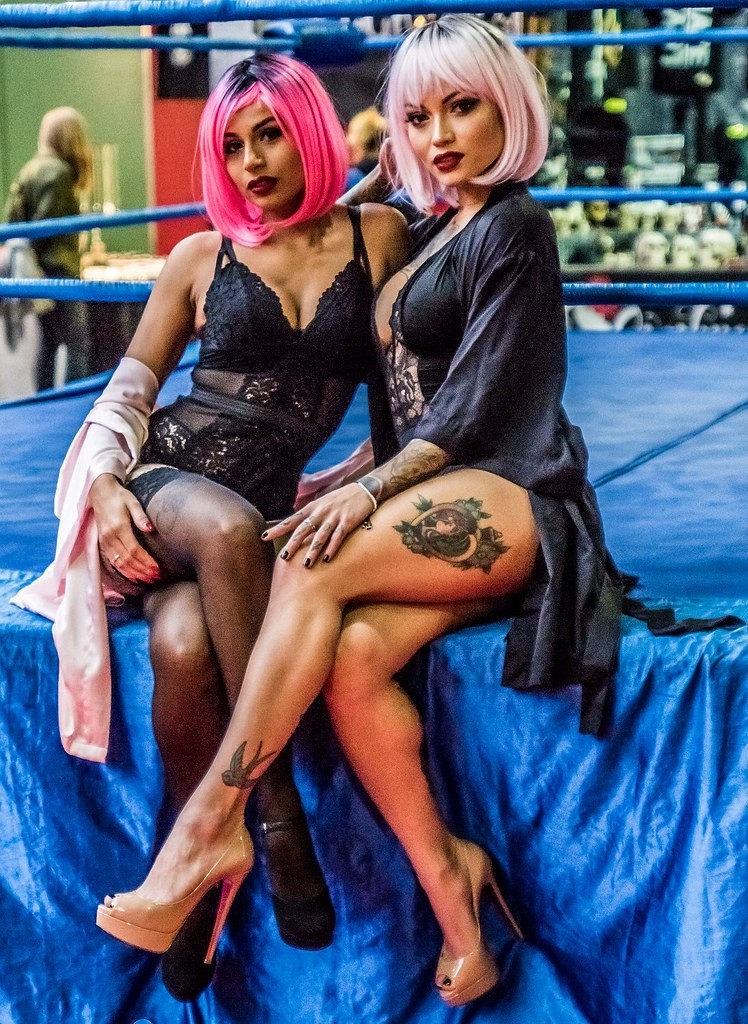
[303,516,317,533]
[109,555,122,567]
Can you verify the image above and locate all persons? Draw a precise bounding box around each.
[540,180,747,336]
[98,13,629,1009]
[0,104,96,395]
[79,56,413,1006]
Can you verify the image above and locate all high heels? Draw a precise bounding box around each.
[161,877,219,1001]
[254,818,337,949]
[434,837,521,1008]
[95,822,254,967]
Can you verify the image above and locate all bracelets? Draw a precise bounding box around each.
[355,480,377,512]
